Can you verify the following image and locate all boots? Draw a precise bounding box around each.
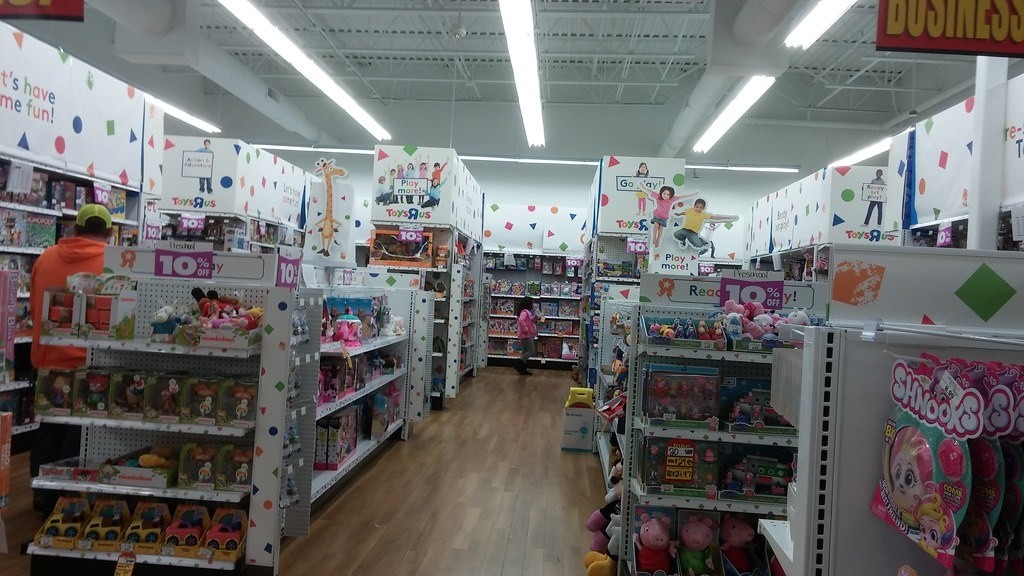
[513,358,525,375]
[522,363,533,375]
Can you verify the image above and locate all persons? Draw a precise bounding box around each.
[197,139,214,194]
[860,169,885,228]
[375,156,450,211]
[30,204,114,523]
[639,181,699,248]
[636,162,649,216]
[674,199,739,258]
[514,296,539,375]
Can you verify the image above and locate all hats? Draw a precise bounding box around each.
[76,204,112,228]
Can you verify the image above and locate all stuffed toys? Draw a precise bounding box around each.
[150,287,264,346]
[650,299,810,354]
[584,432,623,576]
[611,346,628,384]
[632,513,785,576]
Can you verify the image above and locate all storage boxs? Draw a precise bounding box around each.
[488,280,582,360]
[632,302,797,576]
[561,405,594,452]
[1,173,433,560]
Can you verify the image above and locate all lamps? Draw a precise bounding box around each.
[146,0,858,173]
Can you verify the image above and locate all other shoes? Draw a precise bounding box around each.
[711,256,715,258]
[681,241,687,247]
[701,239,708,245]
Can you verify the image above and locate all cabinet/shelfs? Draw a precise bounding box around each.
[368,221,464,401]
[0,154,143,435]
[582,233,641,389]
[907,202,1024,255]
[449,227,483,394]
[308,287,418,505]
[618,272,828,576]
[482,250,745,363]
[749,243,829,282]
[595,283,640,494]
[24,283,294,576]
